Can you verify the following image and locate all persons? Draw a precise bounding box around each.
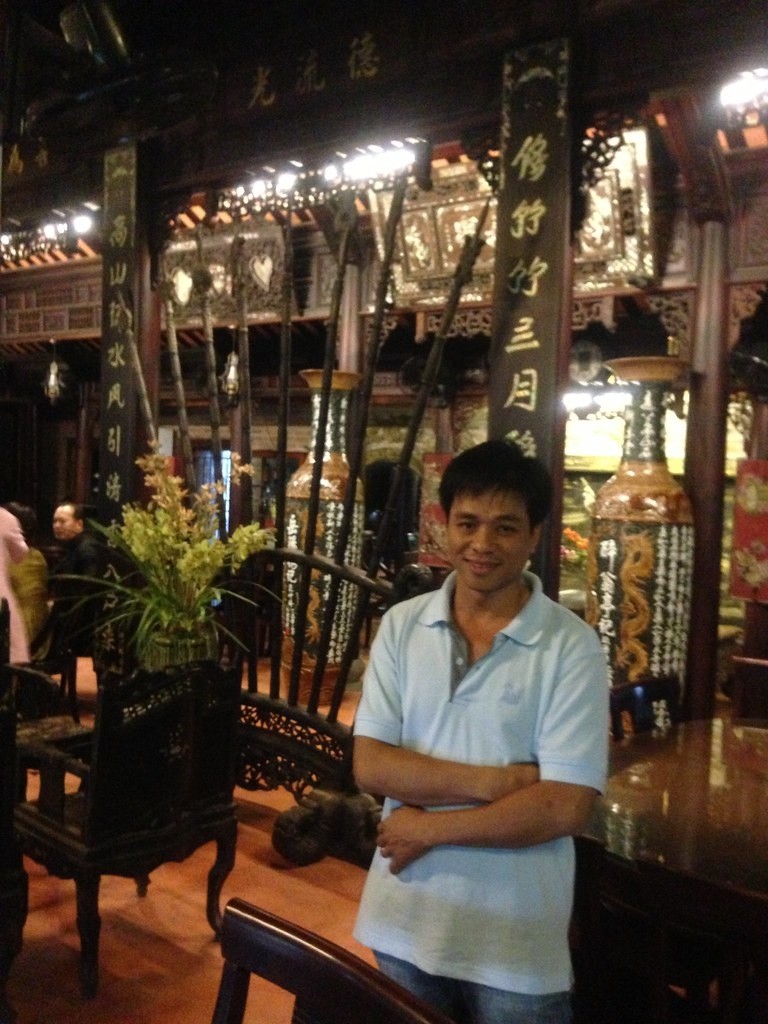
[0,497,102,724]
[348,437,607,1023]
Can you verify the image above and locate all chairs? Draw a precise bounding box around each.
[12,632,252,989]
